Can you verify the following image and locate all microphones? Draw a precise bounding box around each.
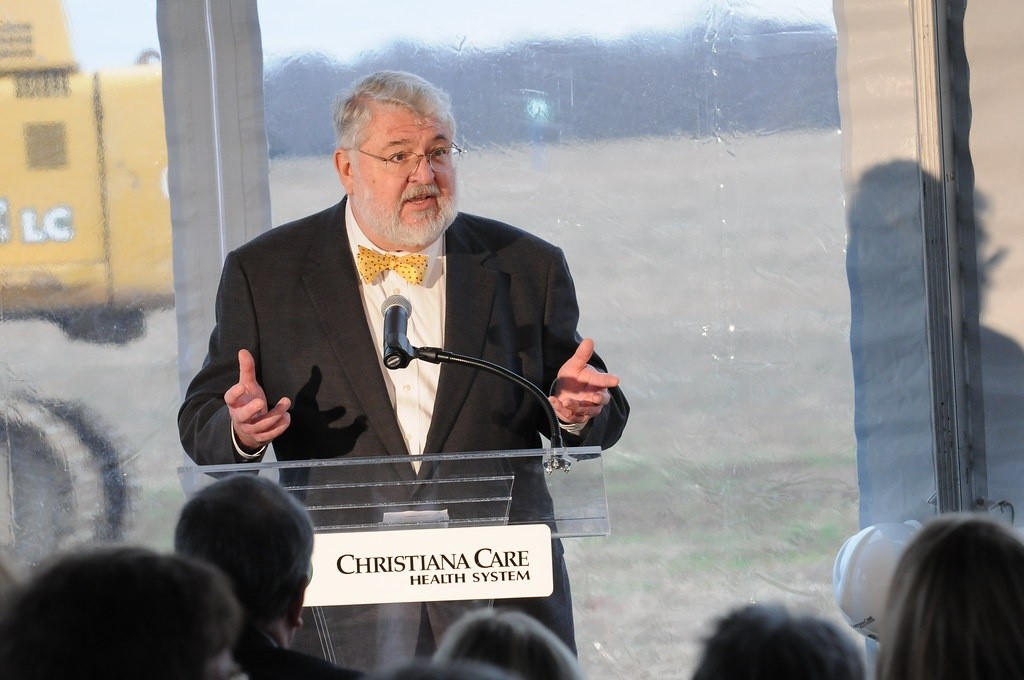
[381,294,412,370]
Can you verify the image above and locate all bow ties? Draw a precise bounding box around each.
[357,244,430,286]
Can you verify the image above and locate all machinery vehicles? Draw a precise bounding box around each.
[2,0,175,346]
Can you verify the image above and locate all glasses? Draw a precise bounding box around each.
[341,140,462,177]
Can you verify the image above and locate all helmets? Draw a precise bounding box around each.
[833,519,925,645]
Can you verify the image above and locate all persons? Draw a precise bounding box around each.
[175,475,367,680]
[875,513,1024,680]
[177,71,629,679]
[0,545,244,680]
[692,605,865,680]
[384,610,574,680]
